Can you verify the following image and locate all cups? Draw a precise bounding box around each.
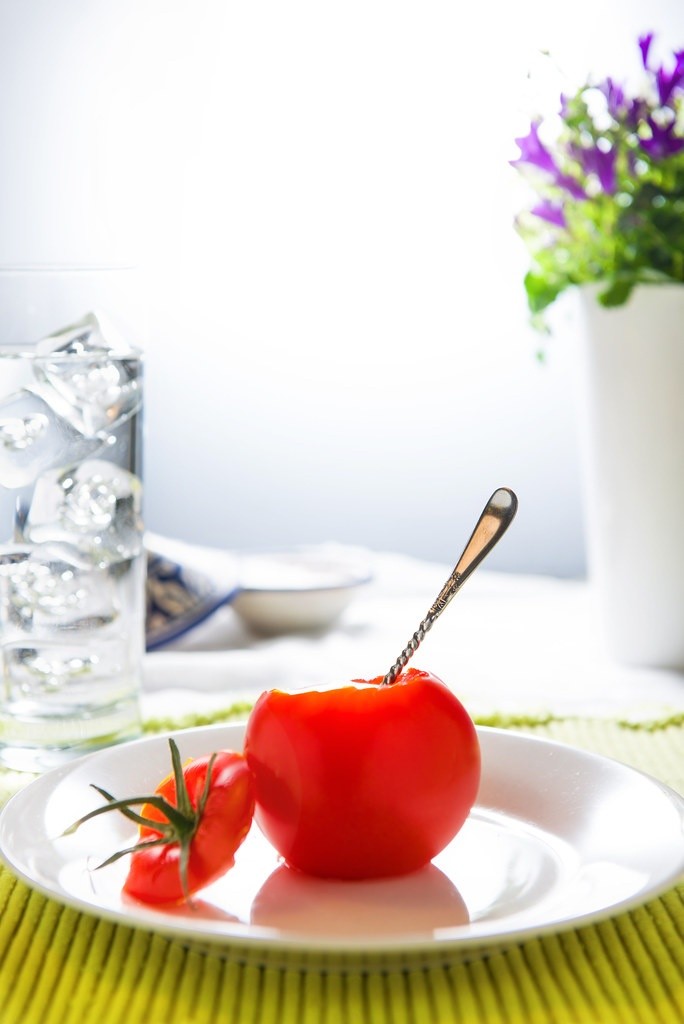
[0,341,149,773]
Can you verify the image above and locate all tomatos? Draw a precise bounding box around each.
[61,737,256,910]
[243,668,482,881]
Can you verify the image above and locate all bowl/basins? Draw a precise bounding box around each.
[226,545,378,636]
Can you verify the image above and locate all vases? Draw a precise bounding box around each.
[574,277,684,672]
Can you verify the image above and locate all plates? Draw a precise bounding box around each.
[0,720,684,957]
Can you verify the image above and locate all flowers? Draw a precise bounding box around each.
[509,21,684,322]
